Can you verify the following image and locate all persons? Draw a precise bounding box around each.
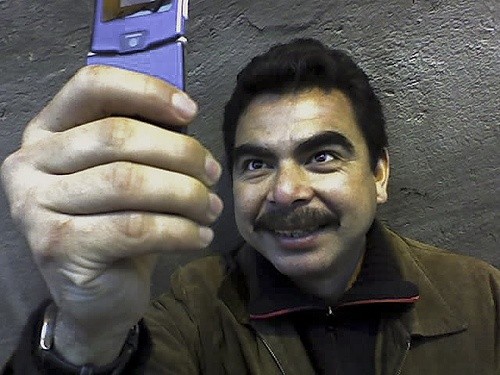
[1,35,500,374]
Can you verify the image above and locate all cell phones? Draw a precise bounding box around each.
[83,0,189,134]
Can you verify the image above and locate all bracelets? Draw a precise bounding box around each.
[33,302,143,375]
